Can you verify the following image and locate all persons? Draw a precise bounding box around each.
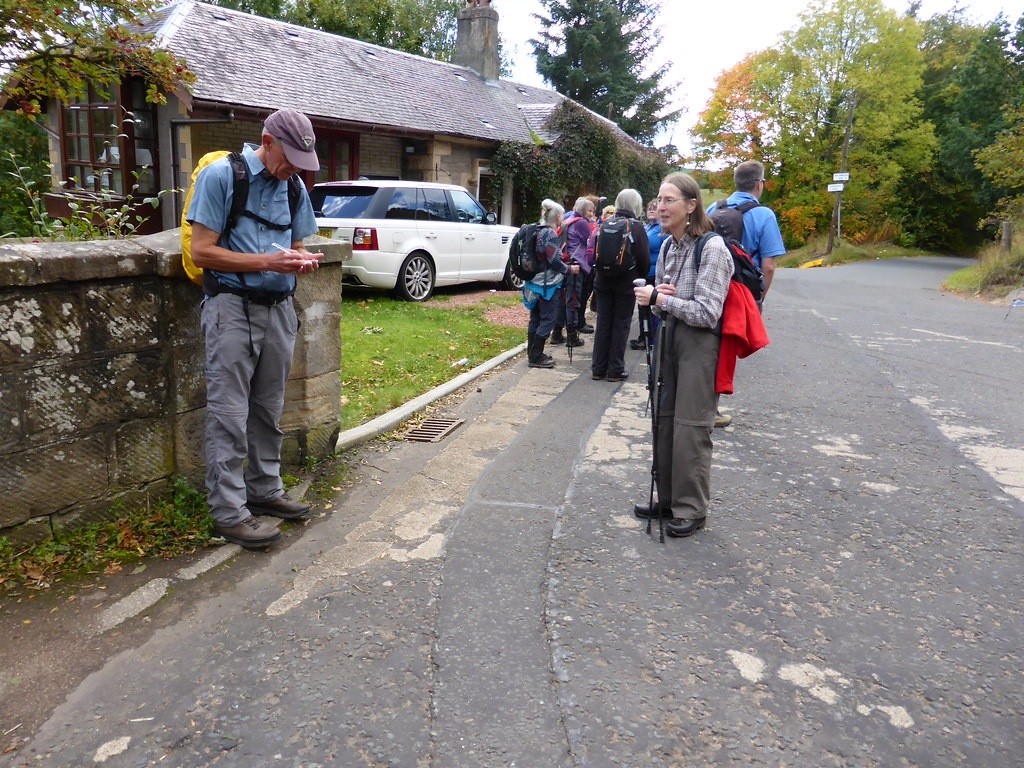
[591,187,651,383]
[186,109,324,547]
[630,199,670,350]
[633,172,735,537]
[550,195,615,348]
[706,160,786,315]
[522,199,579,368]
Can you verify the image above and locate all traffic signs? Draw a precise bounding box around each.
[828,184,844,192]
[832,172,850,181]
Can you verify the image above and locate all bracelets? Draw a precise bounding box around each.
[649,289,658,305]
[661,296,668,311]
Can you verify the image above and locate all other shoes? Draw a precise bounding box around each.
[586,324,593,328]
[577,326,594,334]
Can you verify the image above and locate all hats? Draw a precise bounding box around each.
[264,107,320,171]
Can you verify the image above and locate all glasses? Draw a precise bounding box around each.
[652,197,696,208]
[754,179,766,184]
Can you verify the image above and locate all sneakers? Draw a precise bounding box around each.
[630,337,653,351]
[213,514,282,548]
[665,516,706,537]
[246,492,310,519]
[592,372,608,380]
[608,371,629,382]
[634,502,672,519]
[715,411,732,427]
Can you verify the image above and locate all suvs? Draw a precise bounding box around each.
[307,178,533,302]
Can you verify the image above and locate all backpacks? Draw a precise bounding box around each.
[509,222,554,281]
[710,198,763,258]
[663,231,765,313]
[181,150,301,288]
[555,217,583,263]
[596,215,640,286]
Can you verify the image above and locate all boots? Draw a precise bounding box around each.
[566,331,584,347]
[527,333,556,368]
[551,330,567,344]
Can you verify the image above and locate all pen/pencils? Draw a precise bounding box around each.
[271,242,306,263]
